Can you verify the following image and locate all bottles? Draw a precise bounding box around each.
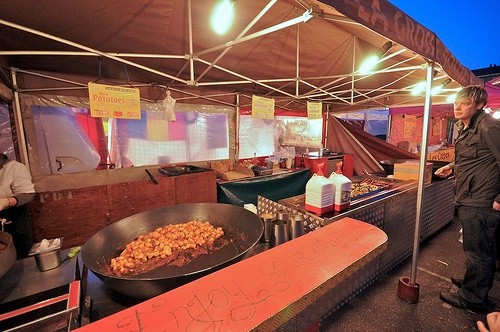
[304,163,334,216]
[252,153,257,166]
[319,142,323,156]
[328,162,352,211]
[280,155,285,168]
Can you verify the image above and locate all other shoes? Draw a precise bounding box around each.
[451,276,464,288]
[440,291,489,313]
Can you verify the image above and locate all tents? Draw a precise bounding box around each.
[0,0,500,289]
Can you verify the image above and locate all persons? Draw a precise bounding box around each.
[477,312,500,332]
[0,153,36,259]
[435,85,500,312]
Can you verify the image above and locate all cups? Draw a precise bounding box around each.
[259,214,273,242]
[270,220,288,249]
[289,217,304,240]
[277,209,290,227]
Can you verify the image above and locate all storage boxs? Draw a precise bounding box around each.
[431,148,455,163]
[395,161,433,185]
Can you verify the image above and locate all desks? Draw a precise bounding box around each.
[217,168,310,207]
[296,151,353,182]
[278,174,454,321]
[27,163,216,251]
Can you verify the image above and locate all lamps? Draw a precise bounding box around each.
[411,71,437,96]
[359,41,393,74]
[209,0,236,36]
[430,79,451,95]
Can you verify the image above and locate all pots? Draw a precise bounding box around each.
[379,159,454,181]
[82,203,264,298]
